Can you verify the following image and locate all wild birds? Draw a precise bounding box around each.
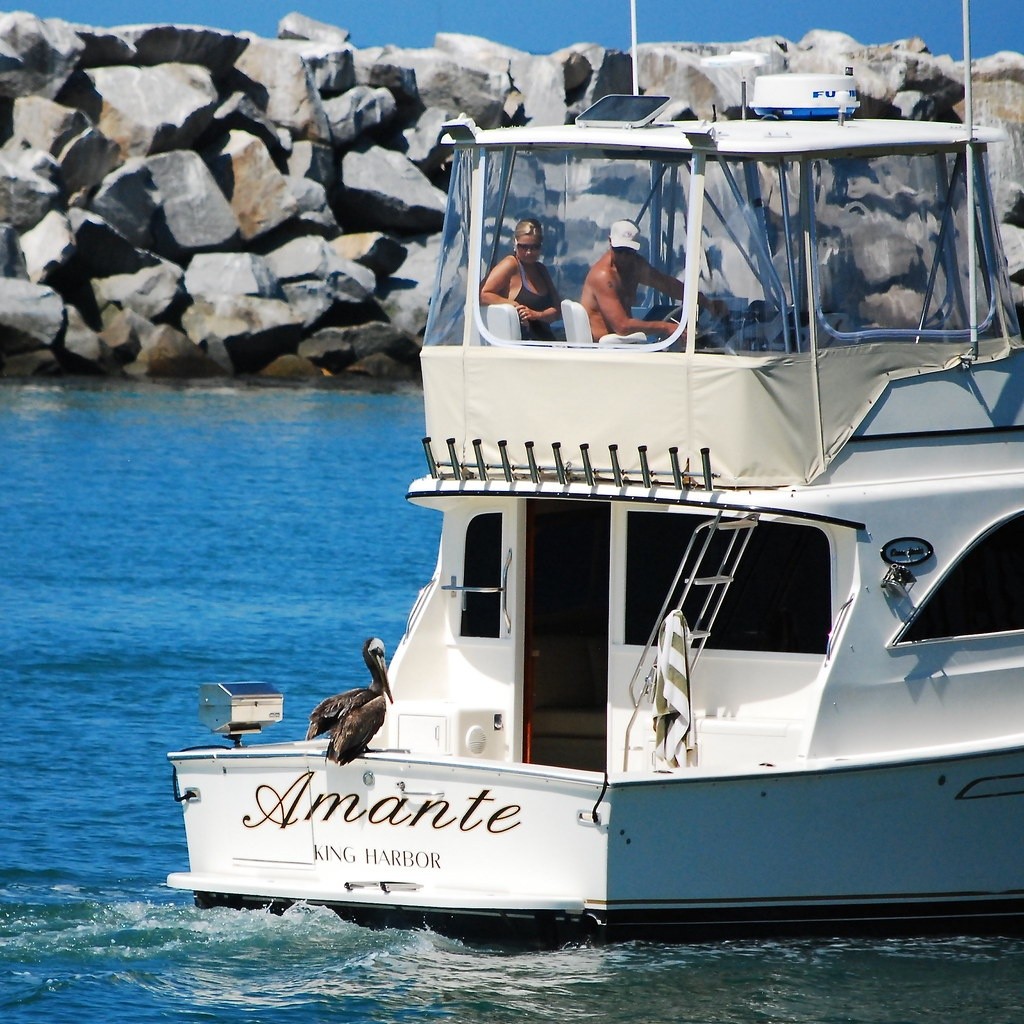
[304,636,396,768]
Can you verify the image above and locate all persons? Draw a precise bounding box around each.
[581,219,729,348]
[479,219,562,341]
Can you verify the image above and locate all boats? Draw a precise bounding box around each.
[166,0,1024,940]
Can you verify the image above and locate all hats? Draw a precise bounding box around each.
[609,219,641,251]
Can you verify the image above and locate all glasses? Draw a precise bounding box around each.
[514,238,542,250]
